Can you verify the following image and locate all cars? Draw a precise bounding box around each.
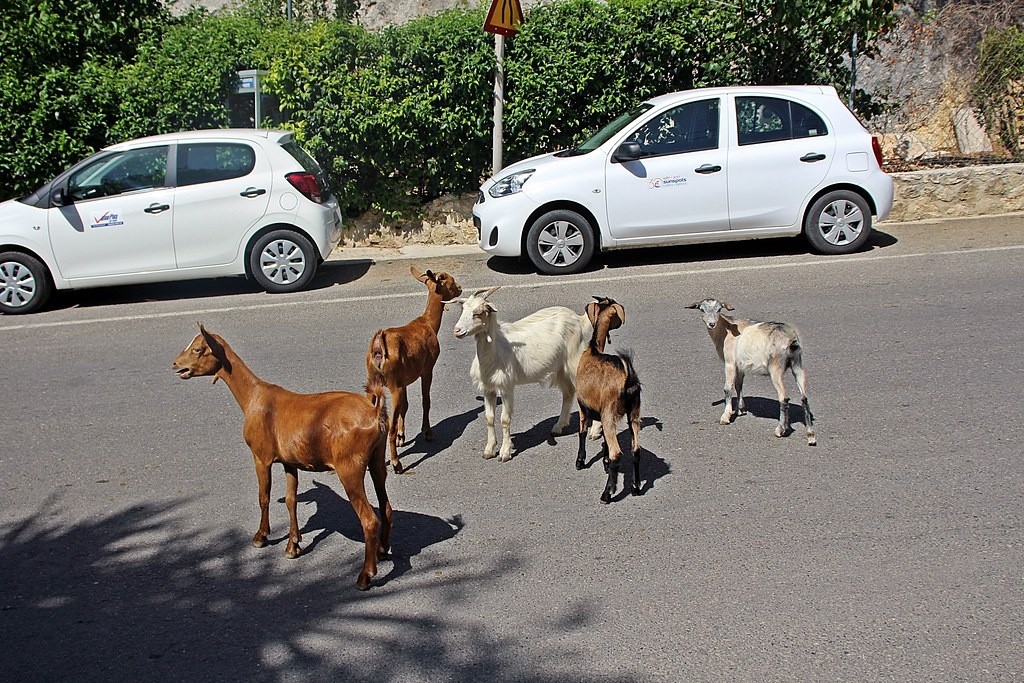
[0,127,347,316]
[470,81,899,280]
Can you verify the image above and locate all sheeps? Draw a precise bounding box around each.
[576,296,641,504]
[367,266,462,474]
[172,321,393,591]
[441,286,603,463]
[685,299,817,446]
